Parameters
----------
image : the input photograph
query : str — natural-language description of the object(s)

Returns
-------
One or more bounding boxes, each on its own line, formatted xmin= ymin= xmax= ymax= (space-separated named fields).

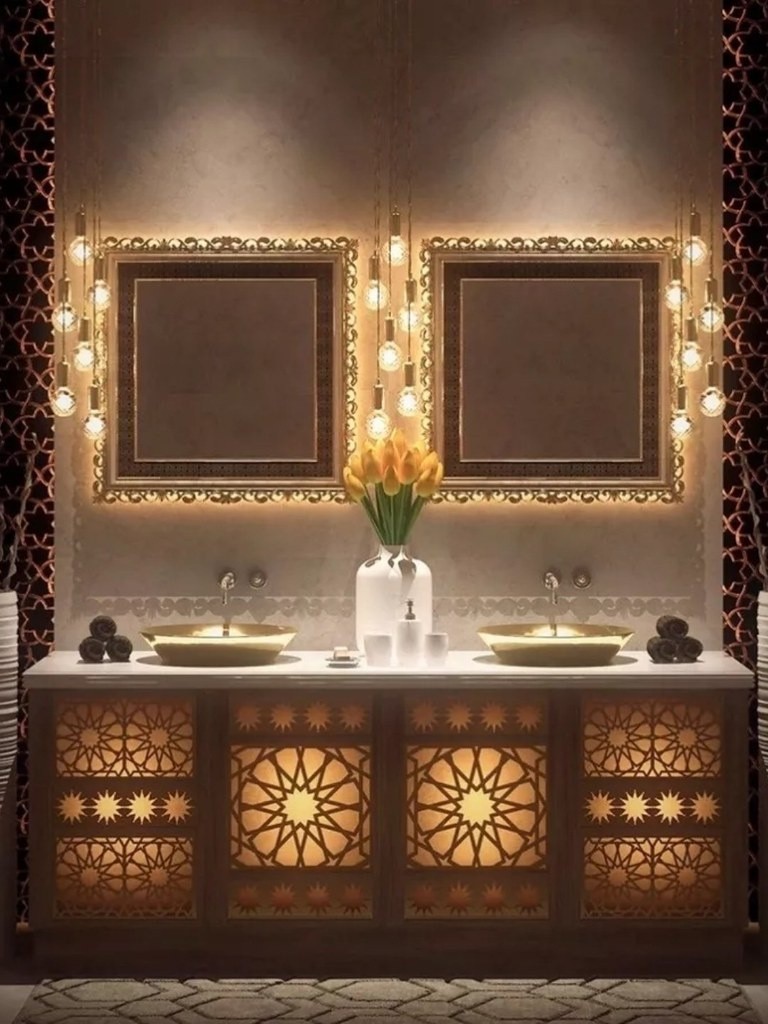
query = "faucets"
xmin=542 ymin=571 xmax=559 ymax=606
xmin=219 ymin=571 xmax=235 ymax=605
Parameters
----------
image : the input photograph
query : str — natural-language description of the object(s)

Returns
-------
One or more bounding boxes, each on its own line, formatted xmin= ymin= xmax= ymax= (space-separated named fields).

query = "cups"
xmin=425 ymin=633 xmax=448 ymax=668
xmin=364 ymin=633 xmax=392 ymax=666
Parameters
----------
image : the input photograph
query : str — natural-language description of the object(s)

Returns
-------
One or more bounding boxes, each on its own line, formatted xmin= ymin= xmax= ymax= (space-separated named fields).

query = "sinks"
xmin=138 ymin=622 xmax=300 ymax=668
xmin=475 ymin=622 xmax=637 ymax=668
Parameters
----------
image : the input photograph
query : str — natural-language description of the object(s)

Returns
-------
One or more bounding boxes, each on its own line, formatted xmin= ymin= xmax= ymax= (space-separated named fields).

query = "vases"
xmin=356 ymin=545 xmax=433 ymax=656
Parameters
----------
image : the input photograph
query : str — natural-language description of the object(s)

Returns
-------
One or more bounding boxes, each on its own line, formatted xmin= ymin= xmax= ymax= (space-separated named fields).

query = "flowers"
xmin=342 ymin=425 xmax=444 ymax=546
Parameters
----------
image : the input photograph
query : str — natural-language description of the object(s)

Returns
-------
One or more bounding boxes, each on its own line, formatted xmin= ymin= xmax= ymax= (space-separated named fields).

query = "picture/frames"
xmin=417 ymin=235 xmax=687 ymax=506
xmin=95 ymin=235 xmax=359 ymax=506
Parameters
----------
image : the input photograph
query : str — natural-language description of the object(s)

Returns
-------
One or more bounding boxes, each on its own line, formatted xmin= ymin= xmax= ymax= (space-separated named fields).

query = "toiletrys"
xmin=395 ymin=599 xmax=424 ymax=666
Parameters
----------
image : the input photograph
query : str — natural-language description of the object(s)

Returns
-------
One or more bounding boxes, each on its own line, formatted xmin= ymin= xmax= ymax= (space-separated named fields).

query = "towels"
xmin=78 ymin=615 xmax=134 ymax=663
xmin=646 ymin=615 xmax=703 ymax=663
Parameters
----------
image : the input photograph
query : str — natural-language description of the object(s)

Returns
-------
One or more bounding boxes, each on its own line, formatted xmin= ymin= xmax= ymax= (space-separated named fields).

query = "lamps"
xmin=51 ymin=0 xmax=114 ymax=439
xmin=664 ymin=0 xmax=725 ymax=436
xmin=362 ymin=0 xmax=424 ymax=440
